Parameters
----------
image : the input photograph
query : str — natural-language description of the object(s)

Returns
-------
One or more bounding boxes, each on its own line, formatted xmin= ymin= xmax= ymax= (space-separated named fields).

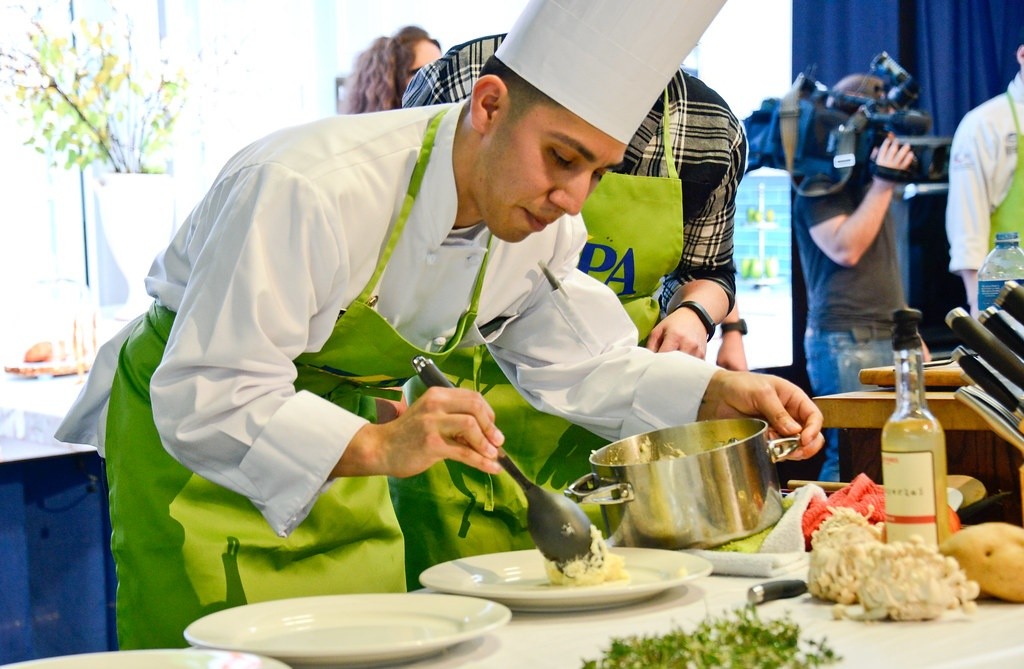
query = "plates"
xmin=420 ymin=544 xmax=714 ymax=612
xmin=183 ymin=593 xmax=512 ymax=668
xmin=1 ymin=647 xmax=294 ymax=668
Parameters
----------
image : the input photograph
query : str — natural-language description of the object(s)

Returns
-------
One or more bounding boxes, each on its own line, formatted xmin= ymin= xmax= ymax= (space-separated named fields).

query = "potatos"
xmin=938 ymin=522 xmax=1024 ymax=604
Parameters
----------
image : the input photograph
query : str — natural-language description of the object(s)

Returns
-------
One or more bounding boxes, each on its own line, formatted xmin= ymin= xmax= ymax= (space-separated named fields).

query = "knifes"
xmin=945 ymin=281 xmax=1024 ymax=460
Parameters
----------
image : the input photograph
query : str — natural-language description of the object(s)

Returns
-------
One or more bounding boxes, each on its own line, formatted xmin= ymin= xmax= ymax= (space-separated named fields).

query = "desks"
xmin=0 ymin=363 xmax=1024 ymax=669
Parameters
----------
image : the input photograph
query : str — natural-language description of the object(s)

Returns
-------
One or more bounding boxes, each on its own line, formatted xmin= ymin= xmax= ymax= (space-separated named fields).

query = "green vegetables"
xmin=576 ymin=603 xmax=845 ymax=669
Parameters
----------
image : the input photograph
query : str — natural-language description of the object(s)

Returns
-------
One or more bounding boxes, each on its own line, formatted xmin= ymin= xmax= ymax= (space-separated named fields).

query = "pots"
xmin=565 ymin=417 xmax=801 ymax=551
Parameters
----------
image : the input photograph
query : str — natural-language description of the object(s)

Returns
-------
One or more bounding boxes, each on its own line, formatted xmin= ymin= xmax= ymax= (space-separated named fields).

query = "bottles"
xmin=880 ymin=308 xmax=948 ymax=550
xmin=978 ymin=232 xmax=1024 ymax=314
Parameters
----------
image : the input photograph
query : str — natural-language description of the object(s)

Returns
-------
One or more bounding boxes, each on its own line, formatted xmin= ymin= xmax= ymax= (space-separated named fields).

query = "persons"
xmin=54 ymin=0 xmax=825 ymax=649
xmin=792 ymin=74 xmax=931 ymax=482
xmin=947 ymin=42 xmax=1024 ymax=318
xmin=343 ymin=26 xmax=748 ymax=591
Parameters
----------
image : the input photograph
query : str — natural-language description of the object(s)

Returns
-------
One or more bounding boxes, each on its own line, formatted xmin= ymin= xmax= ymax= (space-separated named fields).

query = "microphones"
xmin=870 ymin=111 xmax=931 ymax=136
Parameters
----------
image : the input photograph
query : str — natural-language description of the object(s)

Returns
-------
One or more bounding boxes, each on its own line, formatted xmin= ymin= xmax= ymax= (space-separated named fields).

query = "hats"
xmin=495 ymin=0 xmax=727 ymax=146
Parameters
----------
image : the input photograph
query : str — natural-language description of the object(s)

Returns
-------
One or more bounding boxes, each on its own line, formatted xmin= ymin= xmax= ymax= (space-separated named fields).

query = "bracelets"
xmin=674 ymin=300 xmax=715 ymax=341
xmin=722 ymin=318 xmax=748 ymax=335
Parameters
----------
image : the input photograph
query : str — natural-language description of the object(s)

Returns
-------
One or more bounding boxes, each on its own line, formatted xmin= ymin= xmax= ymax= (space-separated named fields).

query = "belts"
xmin=851 ymin=326 xmax=893 ymax=342
